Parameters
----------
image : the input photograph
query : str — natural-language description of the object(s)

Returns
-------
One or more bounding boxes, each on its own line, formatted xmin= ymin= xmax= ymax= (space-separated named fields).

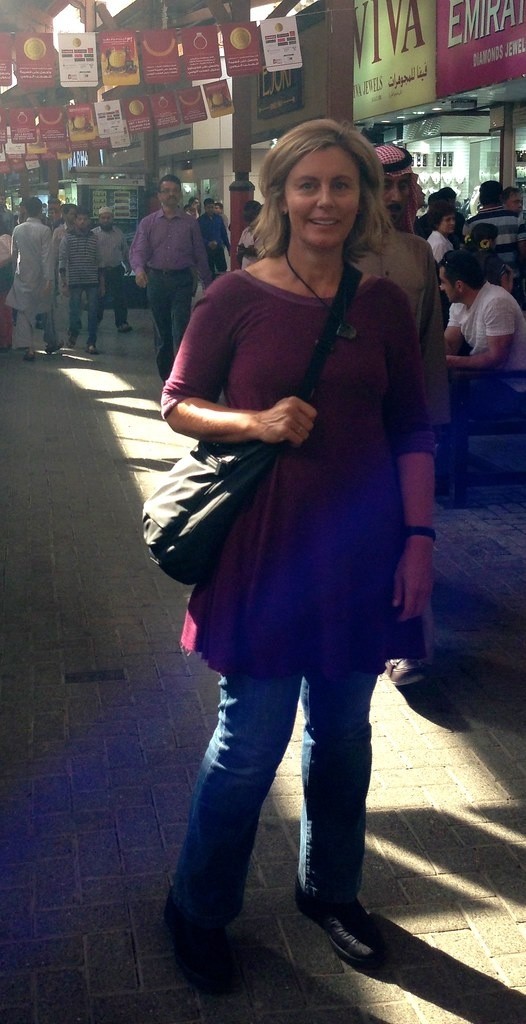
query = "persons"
xmin=461 ymin=181 xmax=526 ymax=310
xmin=415 ymin=187 xmax=465 ymax=240
xmin=6 ymin=197 xmax=63 ymax=360
xmin=503 ymin=187 xmax=523 ymax=212
xmin=236 ymin=200 xmax=262 ymax=270
xmin=161 ymin=119 xmax=436 ymax=993
xmin=214 ymin=203 xmax=229 ymax=229
xmin=92 ymin=207 xmax=132 ymax=332
xmin=130 ymin=174 xmax=212 ymax=387
xmin=184 ymin=197 xmax=200 ymax=220
xmin=197 ymin=198 xmax=230 ymax=279
xmin=52 ymin=203 xmax=77 ymax=348
xmin=437 ymin=250 xmax=526 ymax=509
xmin=59 ymin=205 xmax=99 ymax=354
xmin=426 ymin=200 xmax=456 ymax=263
xmin=467 ymin=224 xmax=513 ymax=292
xmin=349 ymin=148 xmax=451 ymax=687
xmin=0 ymin=192 xmax=65 ymax=352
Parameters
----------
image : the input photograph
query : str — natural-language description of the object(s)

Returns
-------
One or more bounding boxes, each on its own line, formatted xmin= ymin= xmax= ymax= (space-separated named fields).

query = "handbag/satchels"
xmin=142 ymin=439 xmax=273 ymax=583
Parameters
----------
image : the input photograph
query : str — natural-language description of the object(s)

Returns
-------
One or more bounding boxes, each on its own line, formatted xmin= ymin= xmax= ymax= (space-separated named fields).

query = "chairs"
xmin=442 ymin=370 xmax=526 ymax=507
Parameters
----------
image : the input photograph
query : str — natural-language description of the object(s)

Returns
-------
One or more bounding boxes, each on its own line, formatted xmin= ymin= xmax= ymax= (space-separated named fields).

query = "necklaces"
xmin=286 ymin=250 xmax=357 ymax=339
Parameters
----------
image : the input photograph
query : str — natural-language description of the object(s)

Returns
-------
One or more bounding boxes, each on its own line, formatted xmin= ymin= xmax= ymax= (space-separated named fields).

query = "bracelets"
xmin=404 ymin=526 xmax=436 ymax=543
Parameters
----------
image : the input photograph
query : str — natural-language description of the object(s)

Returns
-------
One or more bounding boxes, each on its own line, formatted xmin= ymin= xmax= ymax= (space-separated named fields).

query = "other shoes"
xmin=85 ymin=343 xmax=97 ymax=353
xmin=118 ymin=323 xmax=133 ymax=332
xmin=161 ymin=886 xmax=235 ymax=994
xmin=386 ymin=657 xmax=425 ymax=686
xmin=45 ymin=339 xmax=64 ymax=355
xmin=24 ymin=350 xmax=35 ymax=360
xmin=295 ymin=876 xmax=387 ymax=968
xmin=66 ymin=339 xmax=74 ymax=348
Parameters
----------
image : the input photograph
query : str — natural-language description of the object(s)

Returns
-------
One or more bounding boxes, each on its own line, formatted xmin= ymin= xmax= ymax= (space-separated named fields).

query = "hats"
xmin=99 ymin=207 xmax=112 ymax=215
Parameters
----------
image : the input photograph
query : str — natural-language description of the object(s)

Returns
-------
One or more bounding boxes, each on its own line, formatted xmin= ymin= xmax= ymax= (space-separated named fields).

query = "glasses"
xmin=160 ymin=188 xmax=178 ymax=194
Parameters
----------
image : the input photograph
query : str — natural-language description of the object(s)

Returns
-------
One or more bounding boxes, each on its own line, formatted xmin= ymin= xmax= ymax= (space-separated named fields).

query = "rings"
xmin=297 ymin=427 xmax=302 ymax=433
